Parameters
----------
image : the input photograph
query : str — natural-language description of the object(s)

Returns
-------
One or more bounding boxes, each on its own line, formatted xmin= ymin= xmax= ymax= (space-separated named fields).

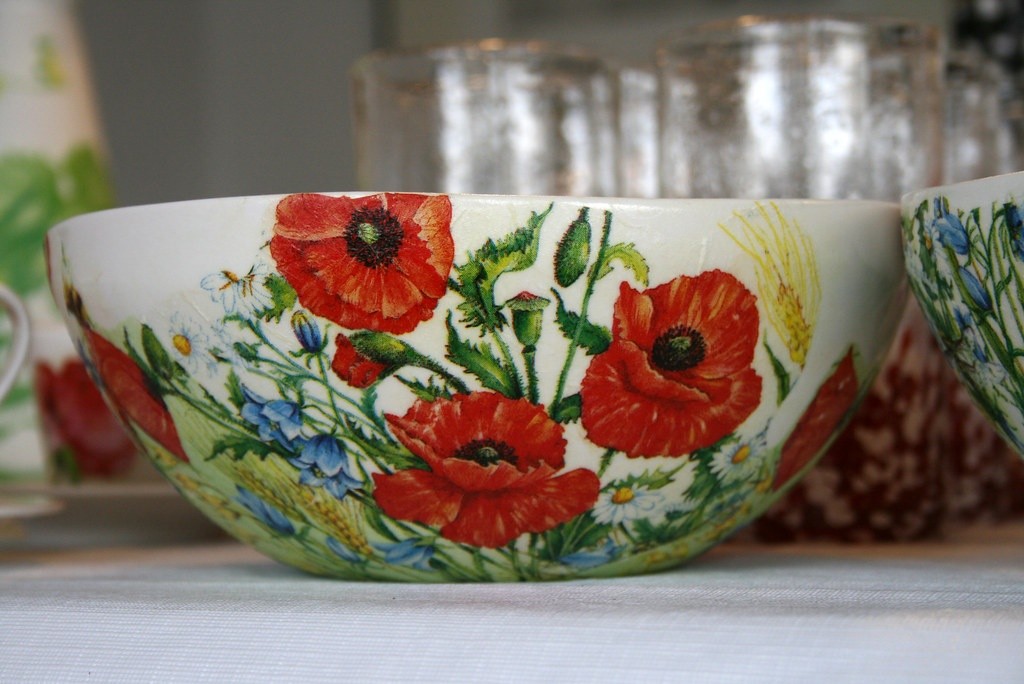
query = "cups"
xmin=350 ymin=15 xmax=1015 ymax=543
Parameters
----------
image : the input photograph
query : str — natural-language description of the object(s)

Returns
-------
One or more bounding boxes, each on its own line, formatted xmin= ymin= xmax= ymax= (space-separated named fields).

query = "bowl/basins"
xmin=42 ymin=190 xmax=913 ymax=585
xmin=900 ymin=169 xmax=1023 ymax=461
